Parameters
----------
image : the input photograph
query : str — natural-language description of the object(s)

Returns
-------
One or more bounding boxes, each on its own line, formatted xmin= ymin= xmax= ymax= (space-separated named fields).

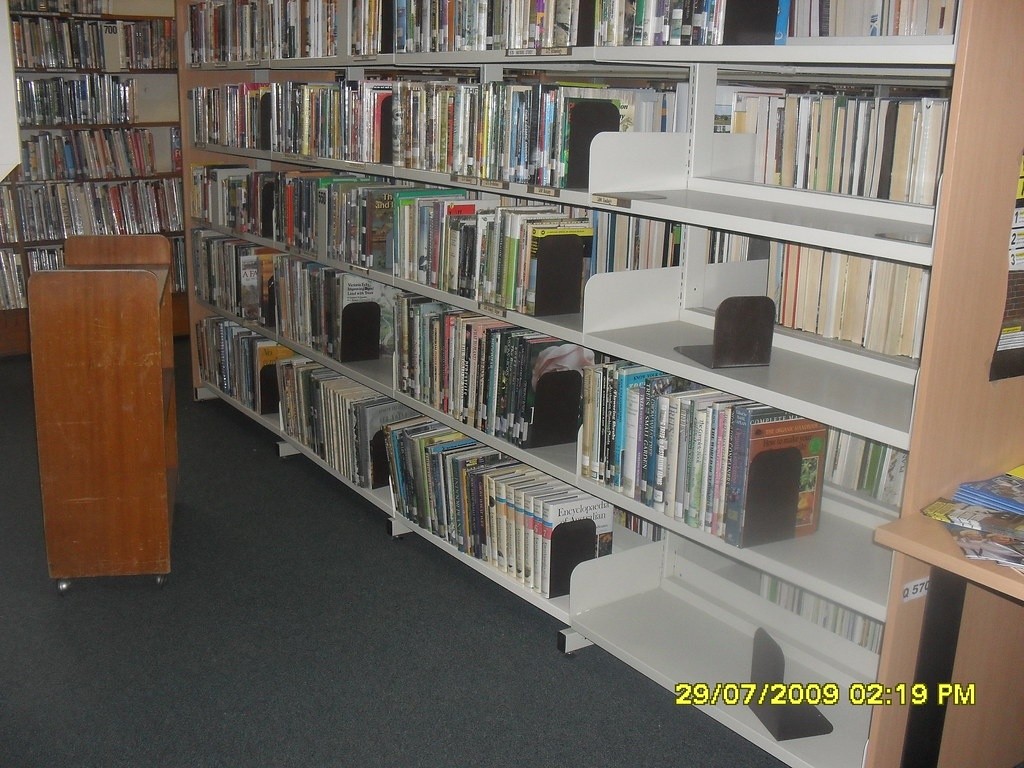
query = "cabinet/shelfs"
xmin=173 ymin=0 xmax=1024 ymax=768
xmin=0 ymin=0 xmax=187 ymax=359
xmin=862 ymin=0 xmax=1024 ymax=768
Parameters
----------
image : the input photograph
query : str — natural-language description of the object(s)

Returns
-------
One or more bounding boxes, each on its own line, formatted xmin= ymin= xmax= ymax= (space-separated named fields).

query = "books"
xmin=0 ymin=0 xmax=1024 ymax=598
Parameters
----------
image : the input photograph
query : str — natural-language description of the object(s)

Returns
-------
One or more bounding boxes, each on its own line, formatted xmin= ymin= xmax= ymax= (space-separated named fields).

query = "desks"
xmin=31 ymin=225 xmax=182 ymax=590
